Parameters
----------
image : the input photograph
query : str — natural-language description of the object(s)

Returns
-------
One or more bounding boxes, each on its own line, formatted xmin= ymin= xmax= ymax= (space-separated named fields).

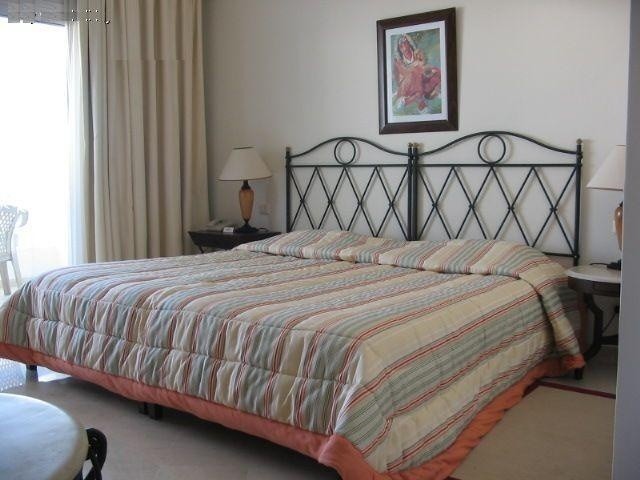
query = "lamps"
xmin=587 ymin=143 xmax=624 ymax=270
xmin=216 ymin=145 xmax=271 ymax=233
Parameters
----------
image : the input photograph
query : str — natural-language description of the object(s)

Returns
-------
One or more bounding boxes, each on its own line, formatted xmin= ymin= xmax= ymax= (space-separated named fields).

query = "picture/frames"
xmin=376 ymin=5 xmax=458 ymax=133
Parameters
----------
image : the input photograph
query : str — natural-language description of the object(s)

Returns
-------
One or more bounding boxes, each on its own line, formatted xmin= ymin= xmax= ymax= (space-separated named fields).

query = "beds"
xmin=24 ymin=132 xmax=581 ymax=475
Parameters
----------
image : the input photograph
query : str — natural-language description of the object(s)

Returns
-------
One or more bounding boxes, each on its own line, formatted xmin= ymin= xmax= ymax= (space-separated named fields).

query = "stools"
xmin=0 ymin=390 xmax=112 ymax=479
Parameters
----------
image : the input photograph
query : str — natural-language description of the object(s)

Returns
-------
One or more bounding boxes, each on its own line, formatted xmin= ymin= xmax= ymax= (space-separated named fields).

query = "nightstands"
xmin=188 ymin=225 xmax=282 ymax=258
xmin=568 ymin=262 xmax=619 ymax=378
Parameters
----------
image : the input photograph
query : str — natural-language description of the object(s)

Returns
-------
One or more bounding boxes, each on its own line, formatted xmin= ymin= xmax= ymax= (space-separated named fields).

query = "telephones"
xmin=203 ymin=218 xmax=236 ymax=232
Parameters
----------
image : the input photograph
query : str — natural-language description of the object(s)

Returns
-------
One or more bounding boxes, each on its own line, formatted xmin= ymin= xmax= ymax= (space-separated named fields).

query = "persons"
xmin=392 ymin=35 xmax=440 ymax=113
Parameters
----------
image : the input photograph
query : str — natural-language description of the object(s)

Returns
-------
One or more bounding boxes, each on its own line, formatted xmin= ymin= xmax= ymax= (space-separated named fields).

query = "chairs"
xmin=0 ymin=204 xmax=28 ymax=296
